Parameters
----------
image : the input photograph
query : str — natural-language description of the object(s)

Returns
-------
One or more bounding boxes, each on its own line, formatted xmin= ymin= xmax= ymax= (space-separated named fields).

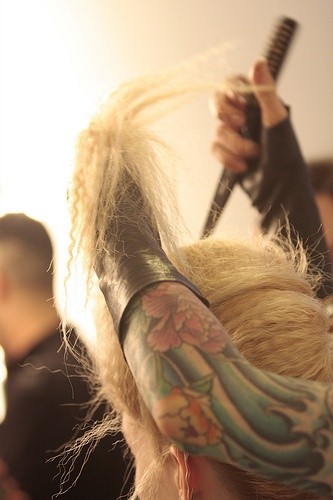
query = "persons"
xmin=86 ymin=57 xmax=333 ymax=498
xmin=92 ymin=240 xmax=333 ymax=500
xmin=0 ymin=211 xmax=134 ymax=500
xmin=306 ymin=158 xmax=333 ymax=259
